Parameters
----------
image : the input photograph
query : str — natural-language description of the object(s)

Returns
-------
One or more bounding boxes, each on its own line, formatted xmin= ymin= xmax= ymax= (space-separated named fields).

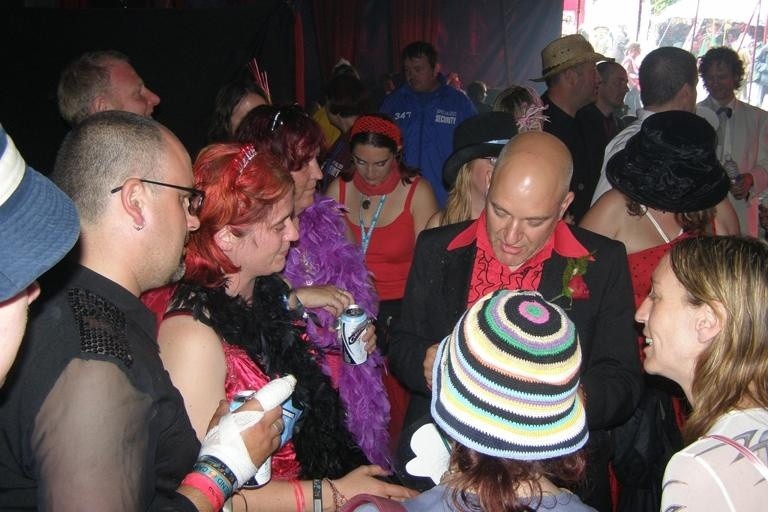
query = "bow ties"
xmin=717 ymin=107 xmax=732 ymax=118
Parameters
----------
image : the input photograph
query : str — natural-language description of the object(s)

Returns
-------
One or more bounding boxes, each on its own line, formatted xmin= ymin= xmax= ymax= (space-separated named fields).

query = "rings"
xmin=338 ymin=287 xmax=345 ymax=299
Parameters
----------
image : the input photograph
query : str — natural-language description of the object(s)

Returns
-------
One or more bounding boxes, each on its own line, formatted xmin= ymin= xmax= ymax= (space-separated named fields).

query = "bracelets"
xmin=325 ymin=477 xmax=348 ymax=511
xmin=221 ymin=491 xmax=248 ymax=512
xmin=291 ymin=477 xmax=322 ymax=512
xmin=181 ymin=453 xmax=237 ymax=512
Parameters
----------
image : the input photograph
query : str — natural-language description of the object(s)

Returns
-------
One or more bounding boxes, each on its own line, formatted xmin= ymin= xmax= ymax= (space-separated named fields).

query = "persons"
xmin=494 ymin=86 xmax=543 ymax=133
xmin=327 ymin=108 xmax=436 ymax=452
xmin=0 ymin=110 xmax=297 ymax=512
xmin=426 ymin=113 xmax=516 ymax=229
xmin=621 ymin=41 xmax=642 ymax=118
xmin=0 ymin=123 xmax=79 ymax=390
xmin=313 ymin=59 xmax=360 ymax=158
xmin=695 ymin=45 xmax=768 ymax=239
xmin=388 ymin=130 xmax=643 ymax=511
xmin=381 ymin=39 xmax=480 ymax=209
xmin=157 ymin=141 xmax=422 ymax=511
xmin=353 ymin=290 xmax=600 ymax=512
xmin=574 ymin=62 xmax=630 ymax=158
xmin=237 ymin=104 xmax=398 ymax=477
xmin=580 ymin=110 xmax=742 ymax=435
xmin=589 ymin=47 xmax=698 ymax=210
xmin=691 ymin=24 xmax=768 ymax=60
xmin=528 ymin=33 xmax=609 ymax=226
xmin=318 ymin=75 xmax=385 ymax=194
xmin=637 ymin=234 xmax=768 ymax=511
xmin=209 ymin=78 xmax=270 ymax=142
xmin=467 ymin=81 xmax=489 ymax=113
xmin=56 ymin=44 xmax=161 ymax=120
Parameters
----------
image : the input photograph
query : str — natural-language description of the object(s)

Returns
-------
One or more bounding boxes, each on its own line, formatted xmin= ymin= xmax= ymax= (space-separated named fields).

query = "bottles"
xmin=339 ymin=304 xmax=368 ymax=366
xmin=228 ymin=390 xmax=272 ymax=489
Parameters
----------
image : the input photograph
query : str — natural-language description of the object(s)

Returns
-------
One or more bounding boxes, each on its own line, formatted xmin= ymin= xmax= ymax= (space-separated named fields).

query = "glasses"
xmin=482 ymin=158 xmax=498 ymax=164
xmin=112 ymin=180 xmax=205 ymax=214
xmin=351 ymin=151 xmax=392 ymax=166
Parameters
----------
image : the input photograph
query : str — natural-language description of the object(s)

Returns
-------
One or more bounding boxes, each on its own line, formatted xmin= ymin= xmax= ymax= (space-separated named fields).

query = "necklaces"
xmin=362 ymin=192 xmax=372 ymax=211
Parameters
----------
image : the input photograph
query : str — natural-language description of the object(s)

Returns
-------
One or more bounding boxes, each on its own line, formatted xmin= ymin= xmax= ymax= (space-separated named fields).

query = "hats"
xmin=606 ymin=110 xmax=730 ymax=212
xmin=529 ymin=35 xmax=615 ymax=82
xmin=431 ymin=290 xmax=589 ymax=460
xmin=442 ymin=111 xmax=518 ymax=191
xmin=0 ymin=122 xmax=81 ymax=304
